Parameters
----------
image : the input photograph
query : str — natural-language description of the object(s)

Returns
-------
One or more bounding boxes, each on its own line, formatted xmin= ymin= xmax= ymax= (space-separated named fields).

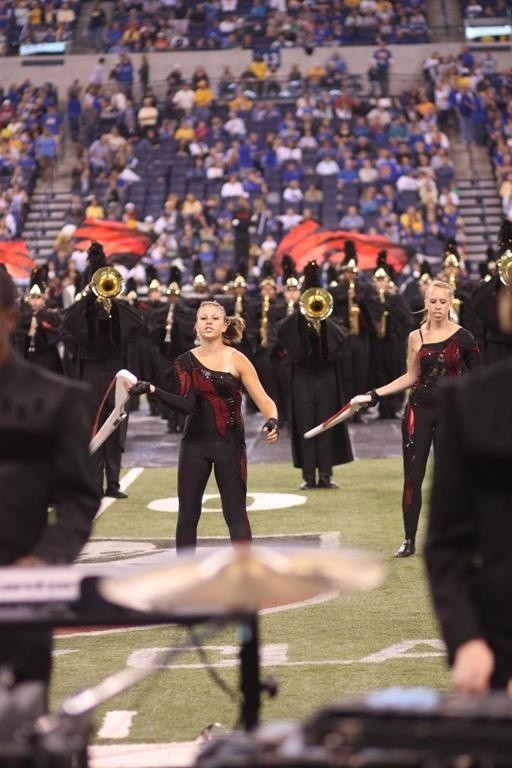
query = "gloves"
xmin=262 ymin=417 xmax=281 ymax=443
xmin=359 ymin=389 xmax=380 ymax=409
xmin=128 ymin=380 xmax=151 ymax=397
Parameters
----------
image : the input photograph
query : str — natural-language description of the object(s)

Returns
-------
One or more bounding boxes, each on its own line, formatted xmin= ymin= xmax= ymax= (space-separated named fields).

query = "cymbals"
xmin=96 ymin=546 xmax=388 ymax=621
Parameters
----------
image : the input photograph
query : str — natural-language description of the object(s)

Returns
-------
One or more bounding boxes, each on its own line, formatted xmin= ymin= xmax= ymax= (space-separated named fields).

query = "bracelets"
xmin=365 ymin=389 xmax=379 ymax=407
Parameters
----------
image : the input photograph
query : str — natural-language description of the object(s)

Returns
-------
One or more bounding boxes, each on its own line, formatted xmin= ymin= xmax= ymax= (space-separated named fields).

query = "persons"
xmin=16 ymin=240 xmax=497 ymax=499
xmin=464 ymin=0 xmax=509 ymax=19
xmin=49 ymin=40 xmax=465 ymax=286
xmin=1 ymin=78 xmax=63 ymax=239
xmin=421 ymin=244 xmax=512 ymax=696
xmin=105 ymin=1 xmax=433 ymax=53
xmin=123 ymin=298 xmax=282 ymax=559
xmin=350 ymin=279 xmax=484 ymax=557
xmin=0 ymin=262 xmax=103 ymax=704
xmin=0 ymin=1 xmax=77 ymax=56
xmin=422 ymin=46 xmax=512 ymax=253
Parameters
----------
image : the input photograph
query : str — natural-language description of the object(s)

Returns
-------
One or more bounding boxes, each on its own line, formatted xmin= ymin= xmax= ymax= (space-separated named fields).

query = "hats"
xmin=342 ymin=254 xmax=460 ymax=286
xmin=27 ymin=254 xmax=113 ymax=296
xmin=248 ymin=254 xmax=339 ymax=293
xmin=124 ymin=263 xmax=247 ymax=297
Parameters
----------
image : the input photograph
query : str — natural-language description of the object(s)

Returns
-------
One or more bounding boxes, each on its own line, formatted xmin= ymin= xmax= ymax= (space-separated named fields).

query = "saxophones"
xmin=347 ymin=279 xmax=360 ymax=335
xmin=260 ymin=295 xmax=269 ymax=349
xmin=376 ymin=290 xmax=389 ymax=340
xmin=447 ymin=275 xmax=461 ymax=326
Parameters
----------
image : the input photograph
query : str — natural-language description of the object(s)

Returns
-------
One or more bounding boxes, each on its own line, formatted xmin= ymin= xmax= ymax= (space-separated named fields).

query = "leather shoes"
xmin=300 ymin=482 xmax=317 ymax=488
xmin=318 ymin=481 xmax=336 ymax=488
xmin=105 ymin=488 xmax=129 ymax=499
xmin=394 ymin=539 xmax=416 ymax=557
